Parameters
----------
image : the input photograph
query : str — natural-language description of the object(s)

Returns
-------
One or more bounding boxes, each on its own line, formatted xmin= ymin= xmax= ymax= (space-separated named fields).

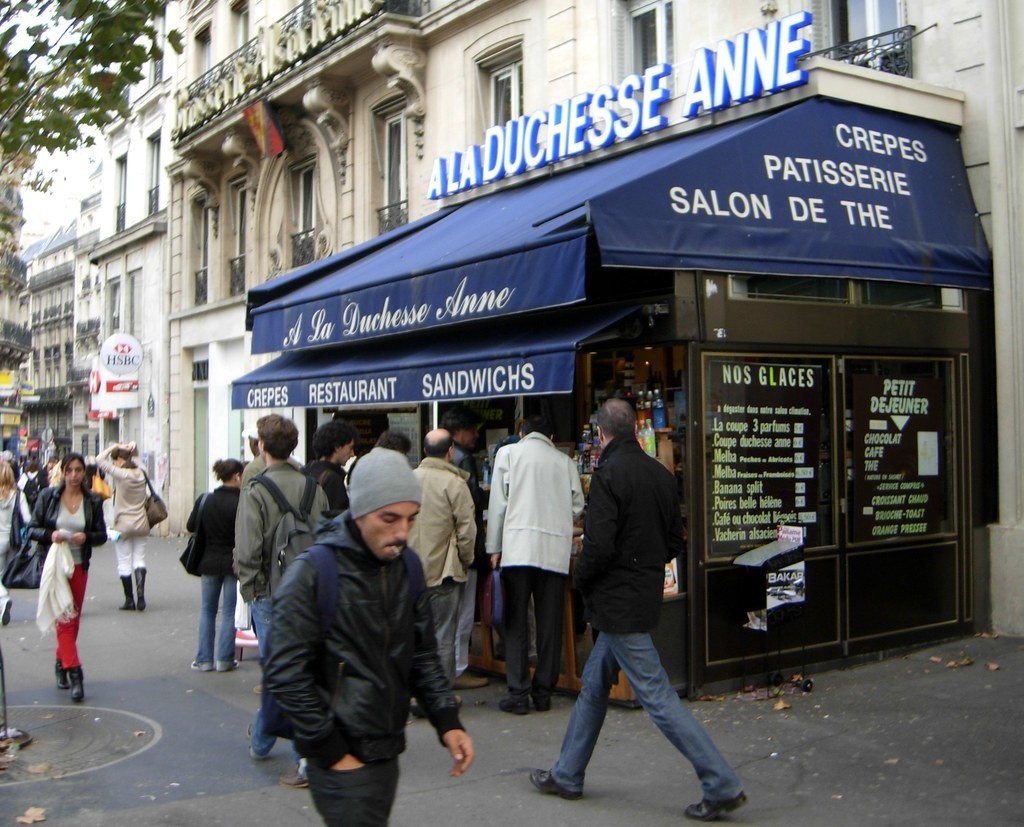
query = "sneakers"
xmin=530 ymin=768 xmax=583 ymax=800
xmin=686 ymin=785 xmax=746 ymax=821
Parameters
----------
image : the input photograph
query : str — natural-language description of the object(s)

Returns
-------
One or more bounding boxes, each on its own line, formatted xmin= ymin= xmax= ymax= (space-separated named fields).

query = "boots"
xmin=136 ymin=568 xmax=147 ymax=610
xmin=56 ymin=659 xmax=71 ymax=689
xmin=120 ymin=574 xmax=135 ymax=610
xmin=69 ymin=666 xmax=84 ymax=701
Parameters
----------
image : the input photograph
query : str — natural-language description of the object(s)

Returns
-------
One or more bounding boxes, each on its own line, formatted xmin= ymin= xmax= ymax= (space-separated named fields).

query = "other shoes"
xmin=217 ymin=660 xmax=240 ymax=671
xmin=247 ymin=718 xmax=268 ymax=760
xmin=1 ymin=598 xmax=12 ymax=624
xmin=191 ymin=660 xmax=212 ymax=671
xmin=279 ymin=772 xmax=311 ymax=788
xmin=452 ymin=671 xmax=489 ymax=688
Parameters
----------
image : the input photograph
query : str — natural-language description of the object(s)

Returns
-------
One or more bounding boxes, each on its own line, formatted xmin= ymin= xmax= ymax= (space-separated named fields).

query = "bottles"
xmin=481 ymin=457 xmax=491 ymax=485
xmin=572 ymin=369 xmax=667 ymax=474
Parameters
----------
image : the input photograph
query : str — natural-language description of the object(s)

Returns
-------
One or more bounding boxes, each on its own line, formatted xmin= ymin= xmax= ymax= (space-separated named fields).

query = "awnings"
xmin=230 ymin=298 xmax=658 ymax=409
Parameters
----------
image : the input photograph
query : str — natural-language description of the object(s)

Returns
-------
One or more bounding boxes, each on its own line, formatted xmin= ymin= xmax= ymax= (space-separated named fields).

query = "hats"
xmin=347 ymin=448 xmax=423 ymax=521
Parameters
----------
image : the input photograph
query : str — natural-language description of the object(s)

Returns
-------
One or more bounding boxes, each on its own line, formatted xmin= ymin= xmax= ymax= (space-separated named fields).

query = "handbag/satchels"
xmin=12 ymin=488 xmax=21 ymax=550
xmin=145 ymin=494 xmax=167 ymax=527
xmin=482 ymin=569 xmax=504 ymax=625
xmin=91 ymin=465 xmax=112 ymax=500
xmin=2 ymin=527 xmax=48 ymax=588
xmin=180 ymin=532 xmax=202 ymax=577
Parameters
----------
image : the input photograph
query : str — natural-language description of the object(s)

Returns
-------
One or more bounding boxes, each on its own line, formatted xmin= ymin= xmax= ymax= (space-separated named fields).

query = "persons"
xmin=186 ymin=459 xmax=245 ymax=672
xmin=231 ymin=415 xmax=489 ymax=789
xmin=529 ymin=398 xmax=747 ymax=820
xmin=0 ymin=448 xmax=59 ymax=548
xmin=264 ymin=447 xmax=475 ymax=827
xmin=84 ymin=454 xmax=105 ymax=506
xmin=26 ymin=453 xmax=107 ymax=700
xmin=485 ymin=415 xmax=584 ymax=714
xmin=0 ymin=462 xmax=31 ymax=626
xmin=95 ymin=441 xmax=150 ymax=611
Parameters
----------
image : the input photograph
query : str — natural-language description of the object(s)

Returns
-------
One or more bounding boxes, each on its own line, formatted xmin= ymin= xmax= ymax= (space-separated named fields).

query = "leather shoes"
xmin=500 ymin=698 xmax=529 ymax=714
xmin=537 ymin=701 xmax=550 ymax=711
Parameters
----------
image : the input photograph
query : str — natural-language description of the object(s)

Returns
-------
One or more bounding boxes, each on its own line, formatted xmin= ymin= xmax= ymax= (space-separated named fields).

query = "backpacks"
xmin=24 ymin=471 xmax=38 ymax=503
xmin=250 ymin=473 xmax=318 ymax=600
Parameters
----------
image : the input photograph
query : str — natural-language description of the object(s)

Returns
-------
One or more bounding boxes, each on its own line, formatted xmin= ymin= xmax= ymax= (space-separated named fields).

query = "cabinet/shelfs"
xmin=564 ymin=348 xmax=685 ymax=493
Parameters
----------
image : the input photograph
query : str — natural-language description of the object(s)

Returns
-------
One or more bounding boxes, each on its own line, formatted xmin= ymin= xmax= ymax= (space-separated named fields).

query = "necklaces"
xmin=66 ymin=498 xmax=80 ymax=508
